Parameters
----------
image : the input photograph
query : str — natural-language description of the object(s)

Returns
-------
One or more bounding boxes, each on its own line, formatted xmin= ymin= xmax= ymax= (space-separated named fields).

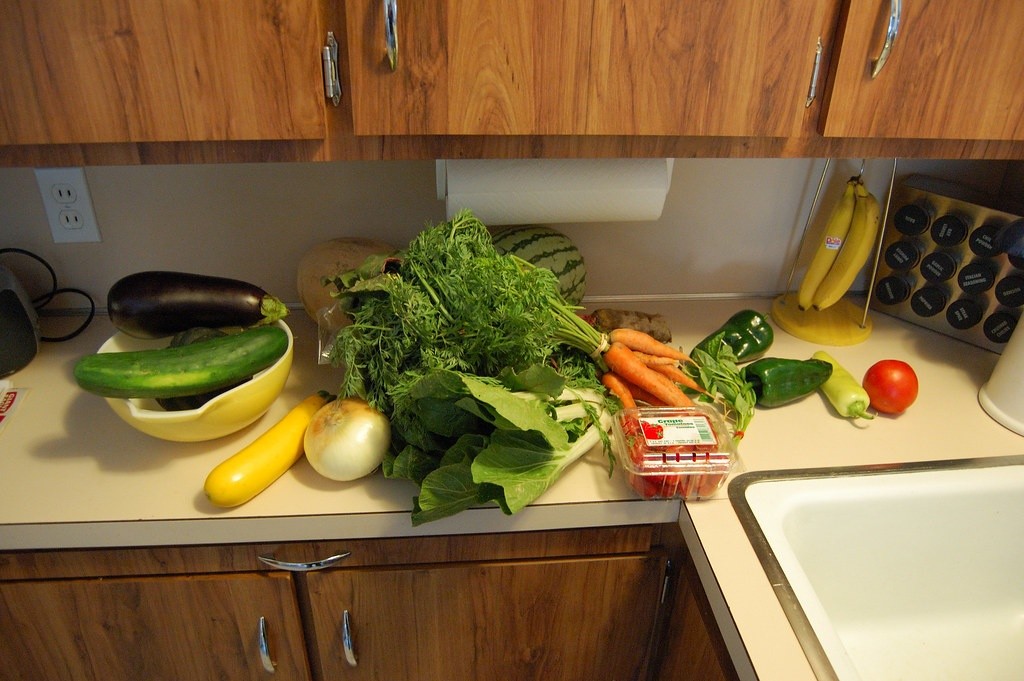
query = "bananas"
xmin=797 ymin=175 xmax=880 ymax=312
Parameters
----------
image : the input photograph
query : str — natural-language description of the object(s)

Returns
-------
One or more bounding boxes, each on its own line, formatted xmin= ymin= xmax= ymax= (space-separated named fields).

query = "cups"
xmin=979 ymin=311 xmax=1024 ymax=434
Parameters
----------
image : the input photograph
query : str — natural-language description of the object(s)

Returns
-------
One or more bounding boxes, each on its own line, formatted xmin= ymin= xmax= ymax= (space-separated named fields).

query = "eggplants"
xmin=106 ymin=270 xmax=291 ymax=339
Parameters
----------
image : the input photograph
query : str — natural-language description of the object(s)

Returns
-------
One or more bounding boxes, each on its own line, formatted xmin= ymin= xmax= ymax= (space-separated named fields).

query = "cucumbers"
xmin=72 ymin=325 xmax=290 ymax=396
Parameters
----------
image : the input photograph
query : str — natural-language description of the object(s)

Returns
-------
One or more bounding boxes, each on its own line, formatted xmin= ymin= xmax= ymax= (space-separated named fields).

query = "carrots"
xmin=599 ymin=327 xmax=724 ymax=418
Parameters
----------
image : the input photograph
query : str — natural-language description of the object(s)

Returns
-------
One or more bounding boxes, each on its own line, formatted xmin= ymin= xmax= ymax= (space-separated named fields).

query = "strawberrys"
xmin=617 ymin=410 xmax=727 ymax=500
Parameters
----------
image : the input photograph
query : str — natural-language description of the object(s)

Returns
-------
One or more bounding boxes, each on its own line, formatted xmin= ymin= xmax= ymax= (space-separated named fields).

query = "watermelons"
xmin=486 ymin=225 xmax=589 ymax=308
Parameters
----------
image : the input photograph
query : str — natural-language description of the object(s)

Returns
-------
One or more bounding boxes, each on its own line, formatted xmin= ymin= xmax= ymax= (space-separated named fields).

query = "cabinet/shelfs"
xmin=0 ymin=0 xmax=1024 ymax=168
xmin=0 ymin=522 xmax=741 ymax=681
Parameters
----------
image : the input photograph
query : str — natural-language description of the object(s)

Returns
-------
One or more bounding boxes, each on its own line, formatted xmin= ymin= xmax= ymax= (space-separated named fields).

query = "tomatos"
xmin=862 ymin=359 xmax=918 ymax=414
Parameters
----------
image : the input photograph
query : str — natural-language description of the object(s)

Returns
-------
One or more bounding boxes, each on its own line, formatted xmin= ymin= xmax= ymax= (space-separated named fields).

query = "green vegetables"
xmin=673 ymin=330 xmax=756 ymax=448
xmin=371 ymin=363 xmax=613 ymax=528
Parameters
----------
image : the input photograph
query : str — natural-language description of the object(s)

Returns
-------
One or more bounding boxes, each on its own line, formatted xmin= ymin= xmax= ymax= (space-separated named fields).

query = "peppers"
xmin=690 ymin=309 xmax=774 ymax=365
xmin=739 ymin=357 xmax=833 ymax=407
xmin=812 ymin=351 xmax=878 ymax=420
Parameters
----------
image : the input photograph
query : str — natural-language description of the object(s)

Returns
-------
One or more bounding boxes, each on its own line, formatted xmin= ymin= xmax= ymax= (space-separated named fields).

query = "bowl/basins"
xmin=97 ymin=319 xmax=294 ymax=442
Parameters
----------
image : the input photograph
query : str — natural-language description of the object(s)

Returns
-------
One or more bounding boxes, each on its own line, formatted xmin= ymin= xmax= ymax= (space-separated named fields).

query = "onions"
xmin=304 ymin=397 xmax=392 ymax=482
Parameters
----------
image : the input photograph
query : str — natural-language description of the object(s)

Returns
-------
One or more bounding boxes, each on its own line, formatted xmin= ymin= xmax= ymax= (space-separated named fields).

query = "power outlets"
xmin=34 ymin=166 xmax=103 ymax=244
